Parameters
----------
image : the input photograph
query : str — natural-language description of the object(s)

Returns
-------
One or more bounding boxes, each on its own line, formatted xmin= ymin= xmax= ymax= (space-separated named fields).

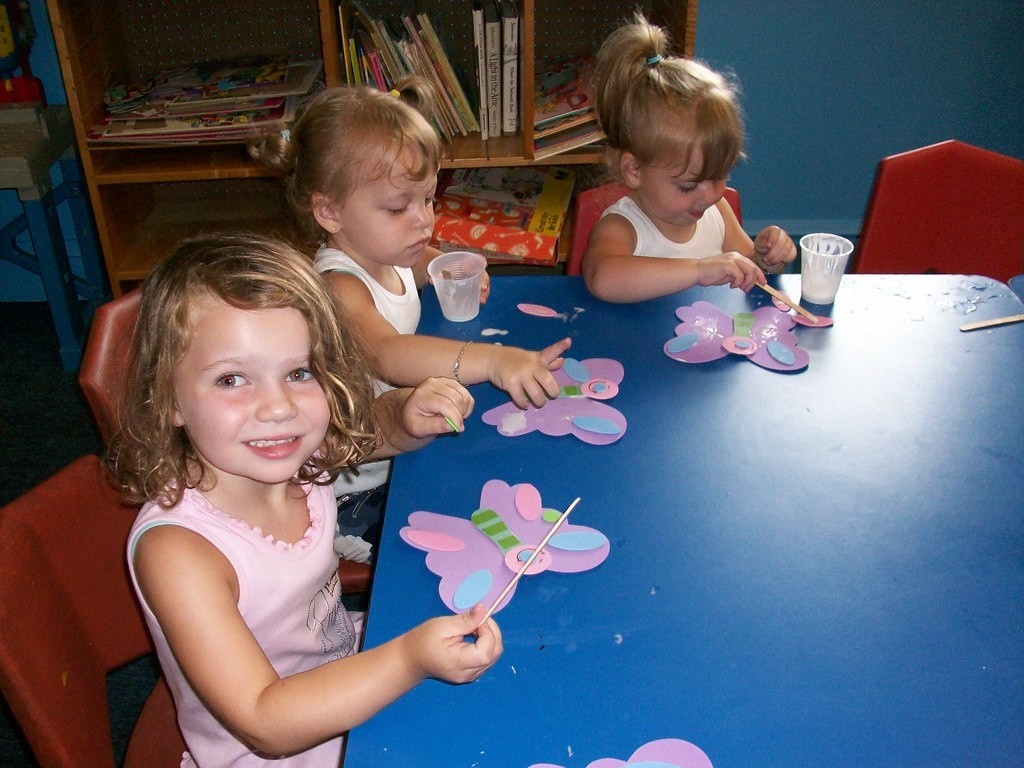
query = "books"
xmin=337 ymin=0 xmax=519 ymax=146
xmin=91 ymin=55 xmax=324 ymax=141
xmin=535 ymin=53 xmax=608 ymax=161
xmin=428 ymin=166 xmax=575 ymax=267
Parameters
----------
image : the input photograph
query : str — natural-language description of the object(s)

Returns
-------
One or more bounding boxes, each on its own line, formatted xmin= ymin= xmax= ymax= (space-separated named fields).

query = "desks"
xmin=0 ymin=103 xmax=110 ymax=376
xmin=343 ymin=271 xmax=1024 ymax=768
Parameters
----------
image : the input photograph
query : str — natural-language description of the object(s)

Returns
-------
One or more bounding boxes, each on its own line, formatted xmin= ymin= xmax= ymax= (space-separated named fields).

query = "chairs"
xmin=564 ymin=182 xmax=742 ymax=276
xmin=0 ymin=453 xmax=189 ymax=768
xmin=77 ymin=288 xmax=374 ymax=597
xmin=846 ymin=138 xmax=1024 ymax=283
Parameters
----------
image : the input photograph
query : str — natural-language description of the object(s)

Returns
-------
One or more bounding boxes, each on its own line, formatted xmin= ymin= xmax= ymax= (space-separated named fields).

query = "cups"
xmin=799 ymin=233 xmax=854 ymax=305
xmin=427 ymin=251 xmax=487 ymax=322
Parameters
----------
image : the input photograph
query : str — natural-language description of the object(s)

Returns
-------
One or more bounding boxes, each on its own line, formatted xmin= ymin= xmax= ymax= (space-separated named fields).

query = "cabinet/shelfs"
xmin=46 ymin=0 xmax=699 ymax=299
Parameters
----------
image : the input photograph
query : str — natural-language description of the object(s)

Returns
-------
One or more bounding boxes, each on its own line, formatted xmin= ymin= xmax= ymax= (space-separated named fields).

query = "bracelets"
xmin=452 ymin=341 xmax=473 ymax=388
xmin=752 ymin=251 xmax=786 ymax=275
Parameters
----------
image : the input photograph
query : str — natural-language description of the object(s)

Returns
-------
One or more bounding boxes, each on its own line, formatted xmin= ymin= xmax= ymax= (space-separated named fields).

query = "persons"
xmin=249 ymin=84 xmax=572 ymax=567
xmin=580 ymin=10 xmax=798 ymax=303
xmin=101 ymin=228 xmax=504 ymax=768
